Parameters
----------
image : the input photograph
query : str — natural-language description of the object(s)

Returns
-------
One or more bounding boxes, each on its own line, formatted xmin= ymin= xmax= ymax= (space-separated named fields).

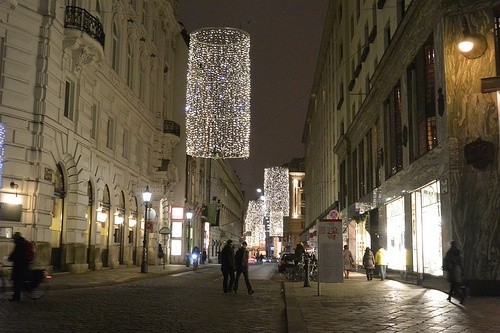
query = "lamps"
xmin=458 ymin=33 xmax=488 ymax=59
xmin=10 ymin=182 xmax=22 ymax=205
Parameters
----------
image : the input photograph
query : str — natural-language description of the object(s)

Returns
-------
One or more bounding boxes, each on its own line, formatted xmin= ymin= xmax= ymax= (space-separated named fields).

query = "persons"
xmin=7 ymin=231 xmax=35 ymax=302
xmin=234 ymin=241 xmax=255 ymax=295
xmin=256 ymin=253 xmax=263 ymax=263
xmin=221 ymin=238 xmax=235 ymax=294
xmin=201 ymin=250 xmax=207 ymax=264
xmin=374 ymin=244 xmax=389 ymax=281
xmin=342 ymin=245 xmax=354 ymax=279
xmin=442 ymin=240 xmax=466 ymax=304
xmin=363 ymin=248 xmax=375 ymax=281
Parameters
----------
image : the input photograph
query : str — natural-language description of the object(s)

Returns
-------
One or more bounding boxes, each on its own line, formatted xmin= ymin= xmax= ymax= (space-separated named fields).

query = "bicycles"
xmin=285 ymin=260 xmax=318 ymax=282
xmin=1 ymin=263 xmax=51 ymax=300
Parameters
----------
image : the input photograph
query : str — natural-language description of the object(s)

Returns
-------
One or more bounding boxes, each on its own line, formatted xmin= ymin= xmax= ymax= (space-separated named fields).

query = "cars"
xmin=277 ymin=252 xmax=296 ymax=272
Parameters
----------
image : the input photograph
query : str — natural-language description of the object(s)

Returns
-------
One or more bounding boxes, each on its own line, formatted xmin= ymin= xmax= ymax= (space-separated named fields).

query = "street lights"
xmin=185 ymin=208 xmax=194 ymax=267
xmin=140 ymin=185 xmax=153 ymax=273
